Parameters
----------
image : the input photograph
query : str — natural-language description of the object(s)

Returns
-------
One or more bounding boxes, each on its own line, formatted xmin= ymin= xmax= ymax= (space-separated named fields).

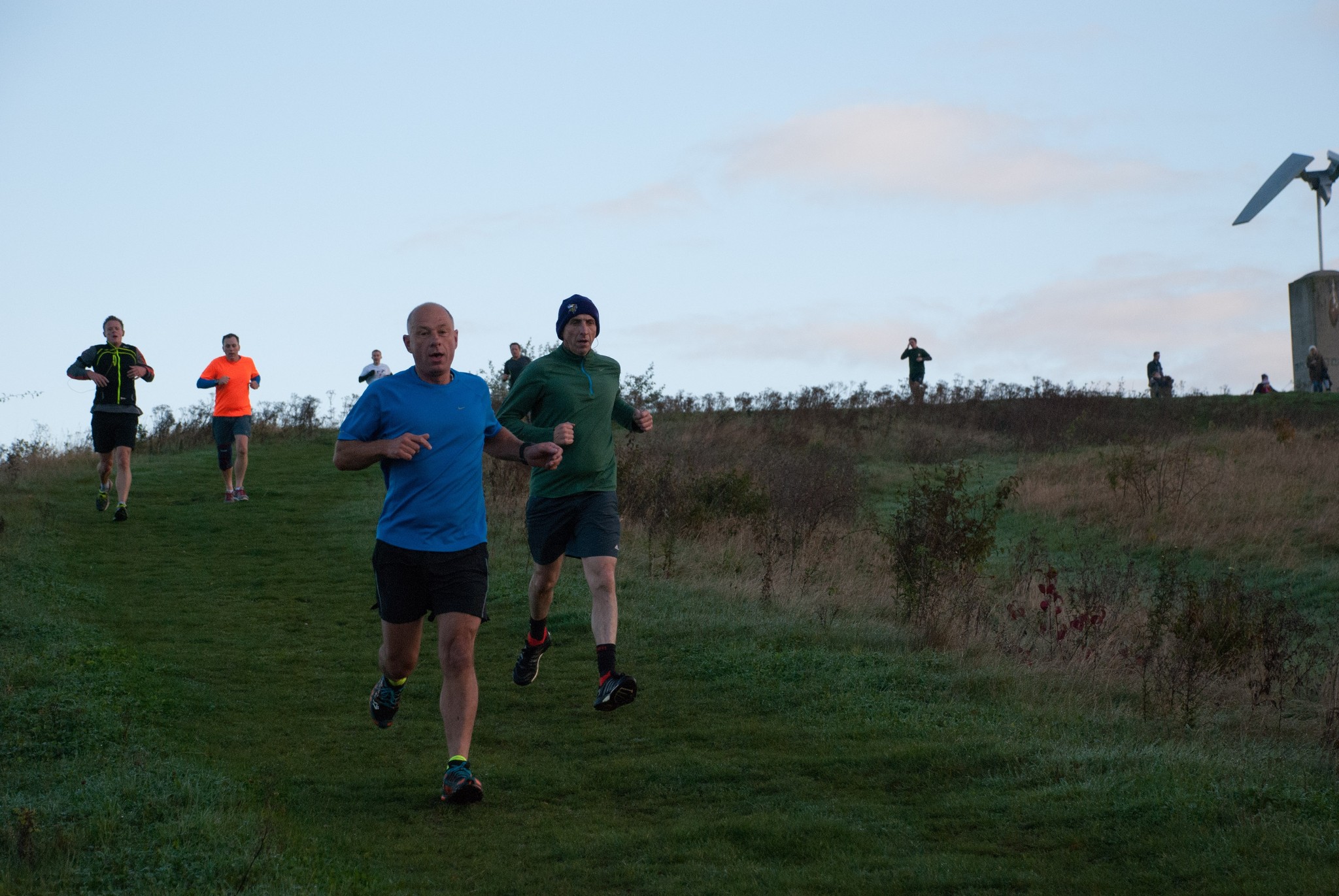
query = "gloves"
xmin=368 ymin=370 xmax=375 ymax=377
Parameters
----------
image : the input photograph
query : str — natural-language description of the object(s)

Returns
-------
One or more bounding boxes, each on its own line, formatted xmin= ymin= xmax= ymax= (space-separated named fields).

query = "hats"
xmin=556 ymin=294 xmax=600 ymax=341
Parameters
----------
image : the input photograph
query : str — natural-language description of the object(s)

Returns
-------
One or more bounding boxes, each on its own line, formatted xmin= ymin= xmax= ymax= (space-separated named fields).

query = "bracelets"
xmin=518 ymin=441 xmax=537 ymax=466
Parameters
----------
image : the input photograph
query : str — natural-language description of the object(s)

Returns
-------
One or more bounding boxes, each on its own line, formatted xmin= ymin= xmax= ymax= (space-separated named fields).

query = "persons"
xmin=196 ymin=332 xmax=261 ymax=506
xmin=66 ymin=315 xmax=155 ymax=522
xmin=358 ymin=349 xmax=393 ymax=386
xmin=502 ymin=342 xmax=531 ymax=392
xmin=497 ymin=294 xmax=653 ymax=714
xmin=1147 ymin=351 xmax=1174 ymax=400
xmin=899 ymin=337 xmax=932 ymax=396
xmin=1254 ymin=374 xmax=1277 ymax=394
xmin=329 ymin=301 xmax=564 ymax=805
xmin=1305 ymin=345 xmax=1333 ymax=393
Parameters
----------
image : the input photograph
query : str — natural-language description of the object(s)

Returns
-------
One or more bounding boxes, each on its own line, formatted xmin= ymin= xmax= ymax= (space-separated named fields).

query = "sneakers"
xmin=594 ymin=670 xmax=638 ymax=712
xmin=234 ymin=486 xmax=249 ymax=501
xmin=440 ymin=759 xmax=484 ymax=804
xmin=223 ymin=485 xmax=236 ymax=503
xmin=369 ymin=675 xmax=408 ymax=728
xmin=513 ymin=631 xmax=553 ymax=686
xmin=113 ymin=503 xmax=128 ymax=521
xmin=96 ymin=479 xmax=113 ymax=511
